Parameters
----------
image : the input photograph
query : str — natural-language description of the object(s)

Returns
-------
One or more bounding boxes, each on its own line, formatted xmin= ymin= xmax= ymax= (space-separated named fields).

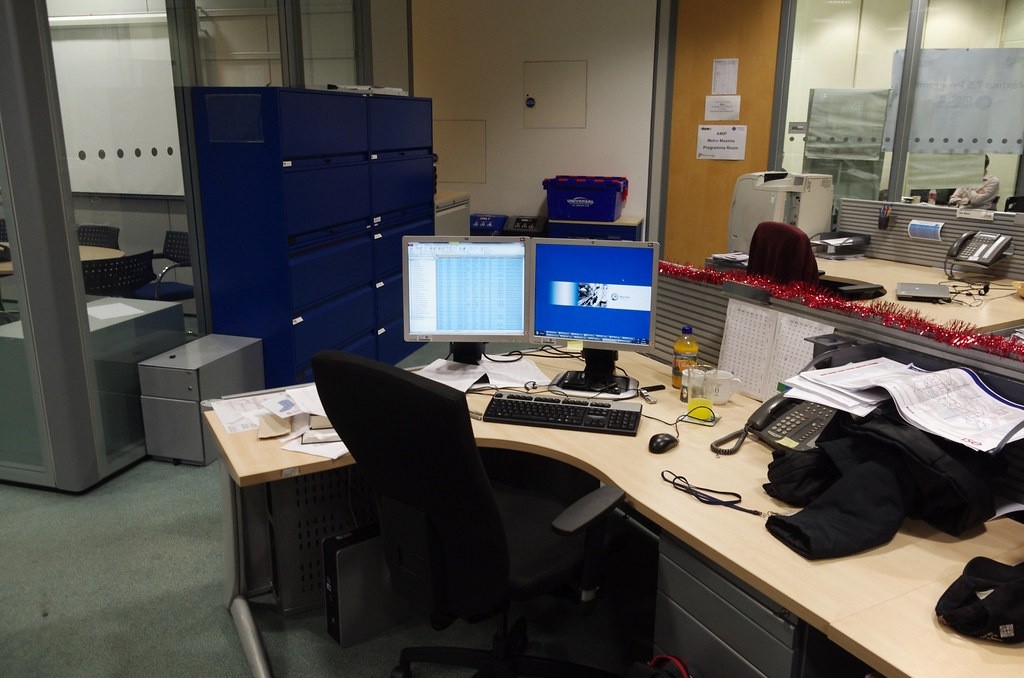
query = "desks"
xmin=0 ymin=242 xmax=125 ymax=324
xmin=705 ymin=250 xmax=1024 ymax=339
xmin=201 ymin=340 xmax=1024 ymax=678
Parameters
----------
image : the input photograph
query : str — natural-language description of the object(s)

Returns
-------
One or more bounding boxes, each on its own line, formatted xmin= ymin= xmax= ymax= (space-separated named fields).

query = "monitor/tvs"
xmin=403 ymin=235 xmax=660 ymax=400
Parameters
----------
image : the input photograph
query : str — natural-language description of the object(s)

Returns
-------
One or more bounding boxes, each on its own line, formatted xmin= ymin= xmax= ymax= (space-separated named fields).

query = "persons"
xmin=948 ymin=154 xmax=1000 ymax=210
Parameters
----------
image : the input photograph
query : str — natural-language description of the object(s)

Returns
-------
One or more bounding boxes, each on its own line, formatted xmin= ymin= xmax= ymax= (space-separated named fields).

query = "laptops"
xmin=896 ymin=283 xmax=951 ymax=303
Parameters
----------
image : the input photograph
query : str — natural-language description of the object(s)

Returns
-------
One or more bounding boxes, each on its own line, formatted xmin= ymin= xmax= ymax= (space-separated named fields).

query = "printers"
xmin=728 ymin=171 xmax=833 ymax=253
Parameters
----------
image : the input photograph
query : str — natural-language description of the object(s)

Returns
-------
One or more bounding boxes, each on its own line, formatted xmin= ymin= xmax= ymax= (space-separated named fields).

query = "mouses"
xmin=649 ymin=433 xmax=679 ymax=454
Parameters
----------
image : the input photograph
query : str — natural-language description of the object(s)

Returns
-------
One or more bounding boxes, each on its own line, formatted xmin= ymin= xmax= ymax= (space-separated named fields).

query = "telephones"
xmin=745 ymin=387 xmax=838 ymax=452
xmin=947 ymin=231 xmax=1013 ymax=266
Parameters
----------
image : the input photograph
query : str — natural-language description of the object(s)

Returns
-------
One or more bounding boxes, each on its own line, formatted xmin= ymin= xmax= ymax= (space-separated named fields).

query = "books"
xmin=777 ymin=356 xmax=1024 ymax=454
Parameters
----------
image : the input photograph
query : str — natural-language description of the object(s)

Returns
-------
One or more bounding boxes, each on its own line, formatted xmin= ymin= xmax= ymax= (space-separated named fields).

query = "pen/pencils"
xmin=879 ymin=204 xmax=892 ymax=216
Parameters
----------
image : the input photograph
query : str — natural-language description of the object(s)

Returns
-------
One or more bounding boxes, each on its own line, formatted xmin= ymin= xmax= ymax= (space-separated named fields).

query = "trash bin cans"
xmin=470 ymin=213 xmax=507 ymax=236
xmin=502 ymin=215 xmax=548 ymax=237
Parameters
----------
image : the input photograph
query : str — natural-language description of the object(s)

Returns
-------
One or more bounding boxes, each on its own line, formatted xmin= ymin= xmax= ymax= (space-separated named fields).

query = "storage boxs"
xmin=139 ymin=334 xmax=265 ymax=465
xmin=541 ymin=174 xmax=628 ymax=222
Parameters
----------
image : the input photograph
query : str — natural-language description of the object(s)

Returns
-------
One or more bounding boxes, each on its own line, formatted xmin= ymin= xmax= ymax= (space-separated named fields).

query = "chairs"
xmin=747 ymin=221 xmax=884 ymax=302
xmin=306 ymin=350 xmax=654 ymax=678
xmin=0 ymin=218 xmax=199 ymax=340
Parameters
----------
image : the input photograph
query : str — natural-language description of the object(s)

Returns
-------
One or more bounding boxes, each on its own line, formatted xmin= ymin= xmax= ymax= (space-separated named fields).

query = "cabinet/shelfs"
xmin=191 ymin=85 xmax=437 ymax=394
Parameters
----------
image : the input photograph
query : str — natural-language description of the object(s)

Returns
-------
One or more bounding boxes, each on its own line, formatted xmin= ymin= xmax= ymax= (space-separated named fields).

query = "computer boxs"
xmin=321 ymin=522 xmax=414 ymax=648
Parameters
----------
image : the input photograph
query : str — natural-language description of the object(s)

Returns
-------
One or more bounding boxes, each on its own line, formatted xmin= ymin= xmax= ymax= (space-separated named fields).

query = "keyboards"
xmin=482 ymin=391 xmax=642 ymax=436
